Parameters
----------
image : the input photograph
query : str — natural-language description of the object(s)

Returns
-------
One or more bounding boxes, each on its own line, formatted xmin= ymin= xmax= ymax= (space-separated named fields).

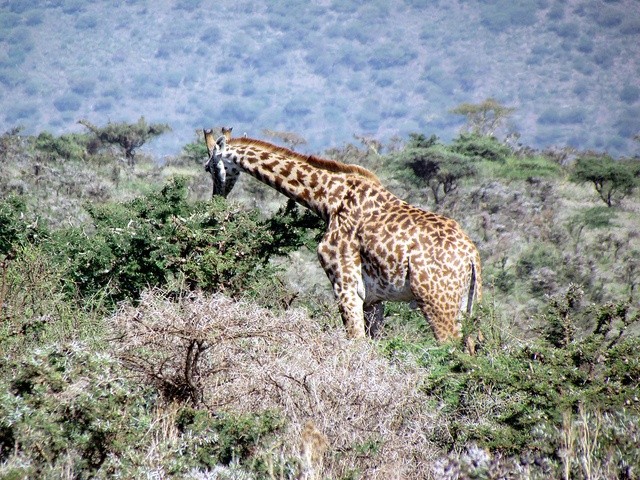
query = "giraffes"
xmin=202 ymin=127 xmax=484 ymax=362
